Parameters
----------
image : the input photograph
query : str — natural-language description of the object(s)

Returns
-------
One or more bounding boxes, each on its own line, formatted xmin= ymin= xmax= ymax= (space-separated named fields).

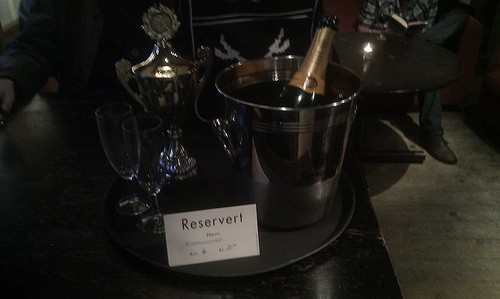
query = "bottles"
xmin=278 ymin=14 xmax=339 ymax=109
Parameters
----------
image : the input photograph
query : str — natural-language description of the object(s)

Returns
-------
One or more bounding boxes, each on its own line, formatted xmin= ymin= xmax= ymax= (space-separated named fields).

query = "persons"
xmin=1 ymin=0 xmax=476 ymax=168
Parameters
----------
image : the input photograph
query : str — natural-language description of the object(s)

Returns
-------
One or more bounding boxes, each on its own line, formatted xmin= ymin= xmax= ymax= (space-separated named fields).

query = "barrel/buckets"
xmin=208 ymin=56 xmax=362 ymax=229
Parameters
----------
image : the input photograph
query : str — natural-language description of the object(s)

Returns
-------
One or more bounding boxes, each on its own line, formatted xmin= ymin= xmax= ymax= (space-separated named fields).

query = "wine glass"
xmin=377 ymin=3 xmax=391 ymax=42
xmin=121 ymin=115 xmax=174 ymax=234
xmin=95 ymin=102 xmax=154 ymax=217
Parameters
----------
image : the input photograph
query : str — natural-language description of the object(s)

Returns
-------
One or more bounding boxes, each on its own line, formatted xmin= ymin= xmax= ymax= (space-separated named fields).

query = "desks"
xmin=328 ymin=32 xmax=464 ymax=192
xmin=0 ymin=33 xmax=405 ymax=299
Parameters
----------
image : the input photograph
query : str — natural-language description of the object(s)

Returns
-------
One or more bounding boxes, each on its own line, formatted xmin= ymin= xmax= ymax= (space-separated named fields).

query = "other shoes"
xmin=420 ymin=128 xmax=457 ymax=165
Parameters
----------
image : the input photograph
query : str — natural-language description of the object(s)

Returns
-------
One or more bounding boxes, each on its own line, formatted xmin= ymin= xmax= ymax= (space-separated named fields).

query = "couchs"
xmin=323 ymin=0 xmax=484 ymax=109
